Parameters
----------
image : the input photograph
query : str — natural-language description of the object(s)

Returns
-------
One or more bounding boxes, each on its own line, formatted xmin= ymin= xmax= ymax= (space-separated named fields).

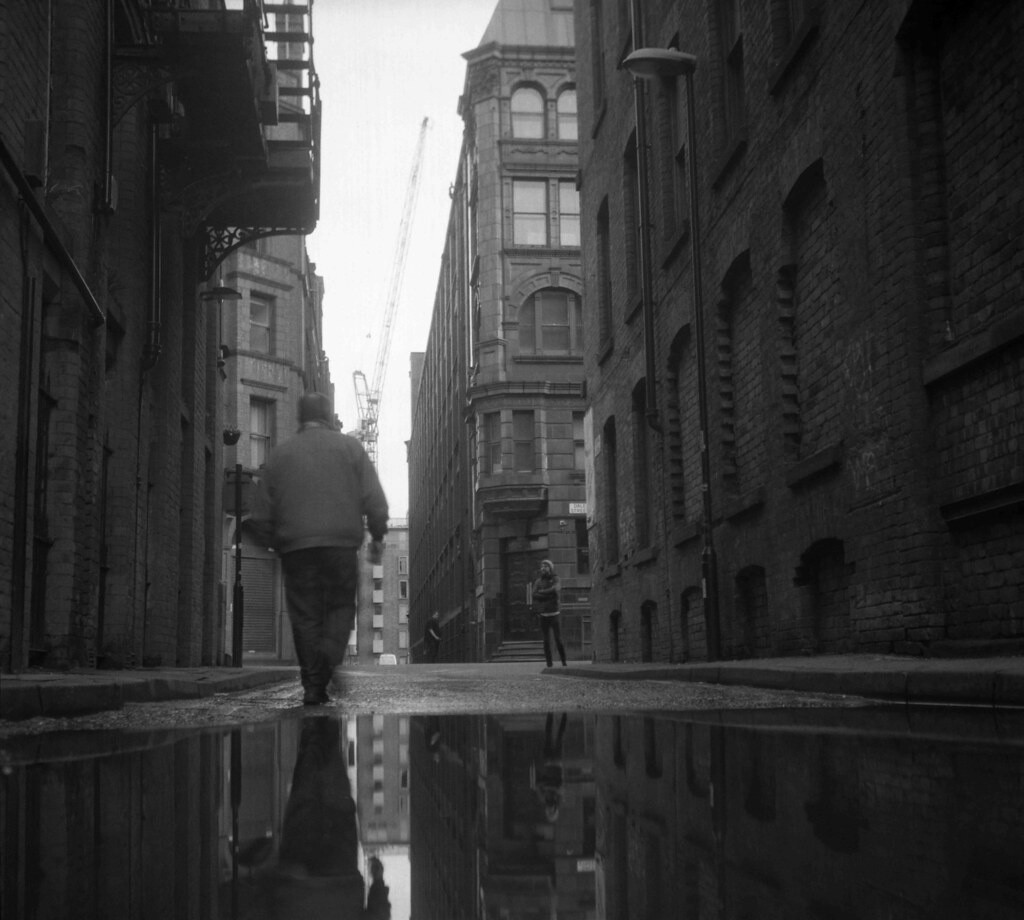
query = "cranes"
xmin=347 ymin=116 xmax=428 ymax=528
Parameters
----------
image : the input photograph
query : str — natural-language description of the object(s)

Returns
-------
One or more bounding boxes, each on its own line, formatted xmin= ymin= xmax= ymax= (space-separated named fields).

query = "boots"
xmin=544 ymin=639 xmax=552 ymax=667
xmin=555 ymin=640 xmax=567 ymax=665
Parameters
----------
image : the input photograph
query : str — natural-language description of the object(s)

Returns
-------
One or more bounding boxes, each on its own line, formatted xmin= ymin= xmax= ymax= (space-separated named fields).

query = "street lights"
xmin=621 ymin=47 xmax=722 ymax=662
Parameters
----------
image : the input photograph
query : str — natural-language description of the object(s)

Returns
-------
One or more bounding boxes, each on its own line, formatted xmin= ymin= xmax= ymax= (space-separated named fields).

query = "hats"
xmin=540 ymin=559 xmax=554 ymax=573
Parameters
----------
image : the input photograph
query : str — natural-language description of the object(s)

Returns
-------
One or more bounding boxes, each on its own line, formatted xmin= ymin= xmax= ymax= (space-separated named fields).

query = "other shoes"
xmin=301 ymin=663 xmax=329 ymax=703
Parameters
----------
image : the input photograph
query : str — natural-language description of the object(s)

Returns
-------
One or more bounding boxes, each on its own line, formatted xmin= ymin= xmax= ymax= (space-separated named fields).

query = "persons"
xmin=532 ymin=560 xmax=567 ymax=668
xmin=251 ymin=393 xmax=389 ymax=703
xmin=426 ymin=610 xmax=442 ymax=663
xmin=529 ymin=713 xmax=567 ymax=821
xmin=222 ymin=715 xmax=391 ymax=920
